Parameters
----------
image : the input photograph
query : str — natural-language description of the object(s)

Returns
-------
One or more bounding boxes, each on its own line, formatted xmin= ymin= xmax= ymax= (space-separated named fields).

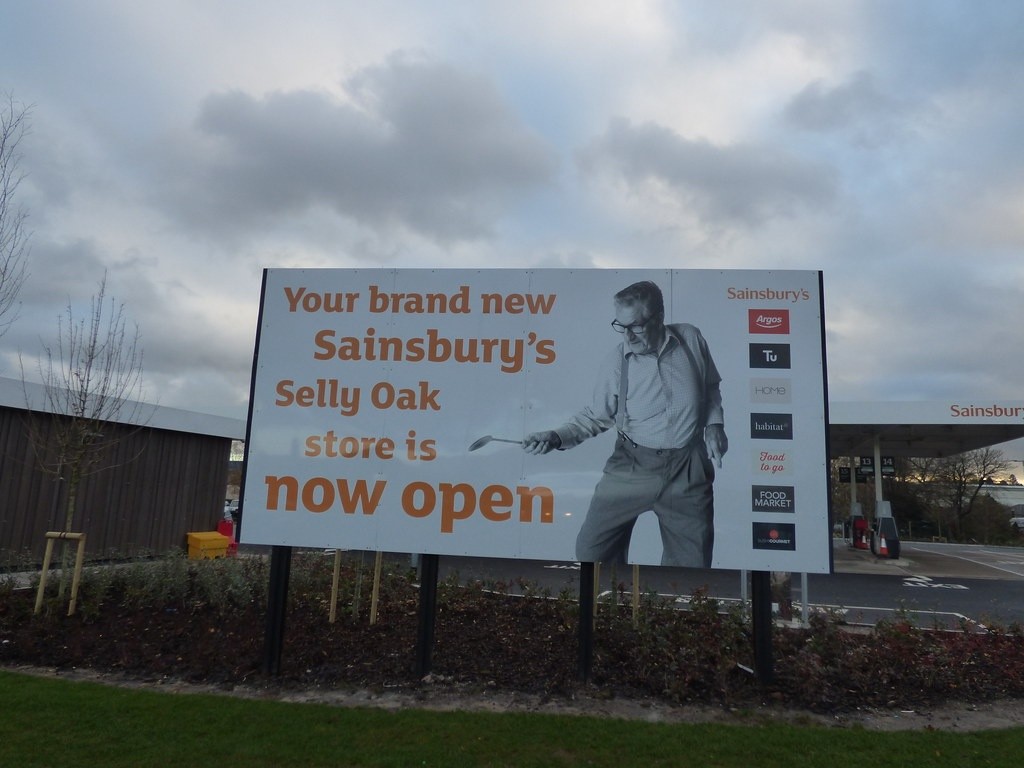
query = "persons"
xmin=523 ymin=281 xmax=728 ymax=569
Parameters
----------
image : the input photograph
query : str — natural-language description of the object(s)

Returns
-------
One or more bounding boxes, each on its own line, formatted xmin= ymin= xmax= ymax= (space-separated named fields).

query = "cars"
xmin=224 ymin=500 xmax=239 ymax=515
xmin=1009 ymin=516 xmax=1024 ymax=530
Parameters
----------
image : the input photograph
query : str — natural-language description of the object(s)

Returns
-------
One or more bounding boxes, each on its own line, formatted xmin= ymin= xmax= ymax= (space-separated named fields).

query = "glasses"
xmin=611 ymin=313 xmax=655 ymax=334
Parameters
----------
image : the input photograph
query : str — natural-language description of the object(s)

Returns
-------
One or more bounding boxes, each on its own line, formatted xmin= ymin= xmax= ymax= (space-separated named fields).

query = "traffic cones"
xmin=880 ymin=533 xmax=889 ymax=554
xmin=860 ymin=531 xmax=868 ymax=548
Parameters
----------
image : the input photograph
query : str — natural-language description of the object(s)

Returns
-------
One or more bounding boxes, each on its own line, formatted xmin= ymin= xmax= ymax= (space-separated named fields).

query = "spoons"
xmin=468 ymin=436 xmax=523 ymax=451
